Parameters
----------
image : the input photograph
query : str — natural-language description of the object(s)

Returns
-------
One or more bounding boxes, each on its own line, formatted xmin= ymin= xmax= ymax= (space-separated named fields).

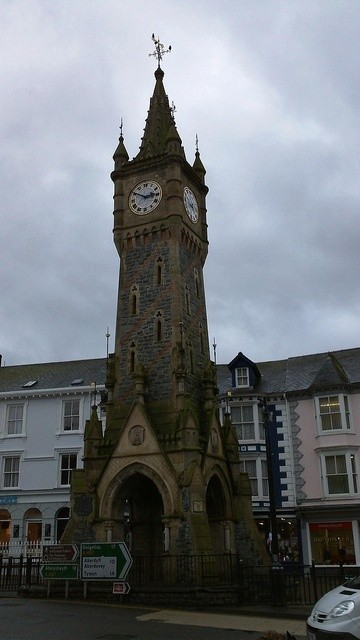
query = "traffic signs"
xmin=41 ymin=544 xmax=79 ymax=562
xmin=113 ymin=582 xmax=130 ymax=594
xmin=80 ymin=542 xmax=133 ymax=581
xmin=39 ymin=564 xmax=78 ymax=579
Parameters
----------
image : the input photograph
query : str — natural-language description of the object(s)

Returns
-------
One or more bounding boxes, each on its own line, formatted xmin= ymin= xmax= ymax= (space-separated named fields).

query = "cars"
xmin=307 ymin=576 xmax=360 ymax=640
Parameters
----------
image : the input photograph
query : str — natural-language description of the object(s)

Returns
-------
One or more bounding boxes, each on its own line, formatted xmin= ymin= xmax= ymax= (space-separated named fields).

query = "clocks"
xmin=128 ymin=180 xmax=162 ymax=215
xmin=183 ymin=186 xmax=199 ymax=224
